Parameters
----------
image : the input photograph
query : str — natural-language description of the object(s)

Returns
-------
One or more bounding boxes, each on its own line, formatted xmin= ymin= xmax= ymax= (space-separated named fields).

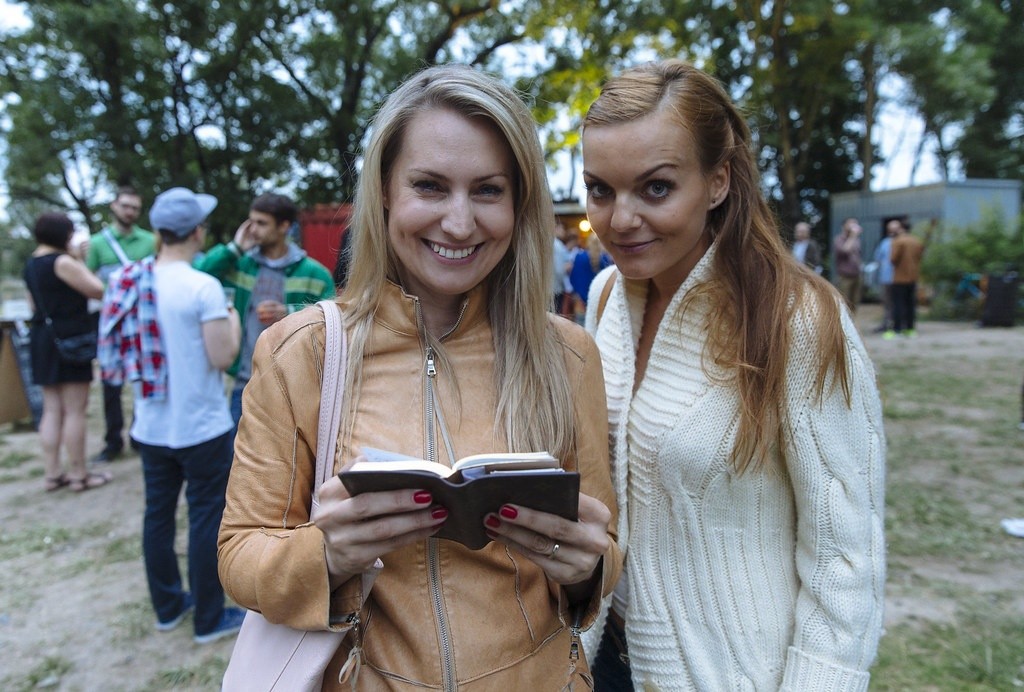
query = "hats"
xmin=148 ymin=186 xmax=219 ymax=238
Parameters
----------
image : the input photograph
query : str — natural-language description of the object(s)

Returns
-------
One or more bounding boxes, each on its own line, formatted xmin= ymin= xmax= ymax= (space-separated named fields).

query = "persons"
xmin=85 ymin=186 xmax=155 ymax=462
xmin=874 ymin=220 xmax=923 ymax=340
xmin=216 ymin=65 xmax=593 ymax=692
xmin=833 ymin=216 xmax=863 ymax=313
xmin=129 ymin=187 xmax=246 ymax=643
xmin=552 ymin=222 xmax=610 ymax=316
xmin=195 ymin=192 xmax=337 ymax=460
xmin=577 ymin=59 xmax=885 ymax=692
xmin=789 ymin=222 xmax=819 ymax=271
xmin=20 ymin=210 xmax=114 ymax=492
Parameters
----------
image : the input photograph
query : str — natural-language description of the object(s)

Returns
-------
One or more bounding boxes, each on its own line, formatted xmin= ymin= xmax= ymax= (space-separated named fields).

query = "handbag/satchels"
xmin=221 ymin=491 xmax=383 ymax=692
xmin=56 ymin=333 xmax=98 ymax=363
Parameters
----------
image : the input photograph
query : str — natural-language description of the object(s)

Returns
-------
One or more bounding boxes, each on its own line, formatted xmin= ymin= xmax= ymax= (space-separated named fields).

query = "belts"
xmin=603 ymin=622 xmax=631 ymax=671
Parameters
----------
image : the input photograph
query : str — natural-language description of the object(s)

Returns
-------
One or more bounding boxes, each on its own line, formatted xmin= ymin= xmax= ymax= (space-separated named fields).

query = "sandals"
xmin=69 ymin=470 xmax=114 ymax=492
xmin=42 ymin=469 xmax=74 ymax=491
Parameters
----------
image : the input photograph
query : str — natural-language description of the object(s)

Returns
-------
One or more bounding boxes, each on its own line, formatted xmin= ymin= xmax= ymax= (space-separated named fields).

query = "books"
xmin=339 ymin=446 xmax=581 ymax=551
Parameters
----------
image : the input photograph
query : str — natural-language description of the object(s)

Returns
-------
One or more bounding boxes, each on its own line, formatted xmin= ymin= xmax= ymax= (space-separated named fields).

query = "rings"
xmin=548 ymin=540 xmax=560 ymax=560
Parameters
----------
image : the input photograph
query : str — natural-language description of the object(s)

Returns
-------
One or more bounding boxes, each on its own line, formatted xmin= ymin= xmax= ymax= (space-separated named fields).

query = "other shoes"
xmin=194 ymin=607 xmax=247 ymax=644
xmin=154 ymin=592 xmax=193 ymax=631
xmin=1000 ymin=517 xmax=1024 ymax=537
xmin=881 ymin=330 xmax=901 ymax=340
xmin=899 ymin=329 xmax=918 ymax=338
xmin=96 ymin=441 xmax=122 ymax=461
